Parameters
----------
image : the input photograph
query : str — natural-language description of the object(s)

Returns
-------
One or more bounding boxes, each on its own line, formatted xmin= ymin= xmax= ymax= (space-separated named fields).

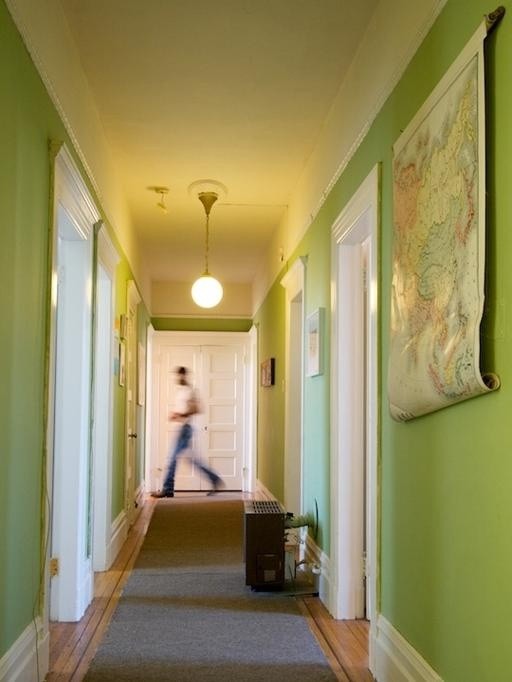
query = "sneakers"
xmin=151 ymin=491 xmax=174 ymax=498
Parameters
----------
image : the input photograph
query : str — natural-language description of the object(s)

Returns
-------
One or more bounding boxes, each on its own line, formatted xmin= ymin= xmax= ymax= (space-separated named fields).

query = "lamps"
xmin=188 ymin=191 xmax=226 ymax=310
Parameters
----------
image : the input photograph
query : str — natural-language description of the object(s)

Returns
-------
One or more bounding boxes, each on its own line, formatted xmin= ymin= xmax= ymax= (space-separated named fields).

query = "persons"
xmin=149 ymin=366 xmax=226 ymax=498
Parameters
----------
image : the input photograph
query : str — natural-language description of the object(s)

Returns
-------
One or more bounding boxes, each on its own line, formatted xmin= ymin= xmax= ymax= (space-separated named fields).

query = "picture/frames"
xmin=306 ymin=306 xmax=325 ymax=379
